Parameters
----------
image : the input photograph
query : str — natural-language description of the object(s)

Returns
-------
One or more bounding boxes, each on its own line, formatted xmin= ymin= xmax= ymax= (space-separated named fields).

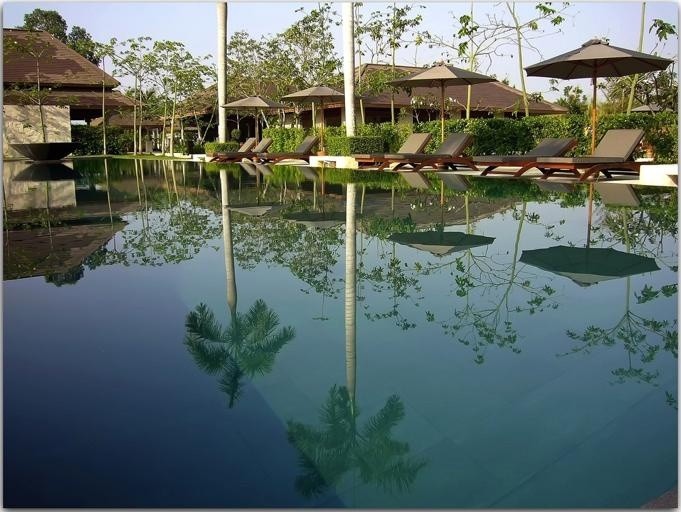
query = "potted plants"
xmin=12 ymin=164 xmax=87 ymax=288
xmin=3 ymin=26 xmax=82 ymax=163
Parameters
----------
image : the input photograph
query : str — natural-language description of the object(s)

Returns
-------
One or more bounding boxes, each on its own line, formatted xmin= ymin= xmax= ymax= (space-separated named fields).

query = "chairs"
xmin=386 ymin=132 xmax=480 ymax=172
xmin=538 ymin=129 xmax=647 ymax=178
xmin=349 ymin=132 xmax=437 ymax=172
xmin=483 ymin=138 xmax=582 ymax=178
xmin=257 ymin=136 xmax=321 ymax=164
xmin=212 ymin=134 xmax=272 ymax=163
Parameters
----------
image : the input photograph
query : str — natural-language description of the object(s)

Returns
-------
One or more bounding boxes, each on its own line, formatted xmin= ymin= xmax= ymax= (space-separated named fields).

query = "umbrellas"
xmin=522 ymin=39 xmax=674 ymax=156
xmin=281 ymin=168 xmax=362 ymax=229
xmin=518 ymin=183 xmax=662 ymax=288
xmin=228 ymin=168 xmax=289 ymax=217
xmin=280 ymin=85 xmax=345 ymax=152
xmin=220 ymin=96 xmax=290 ymax=147
xmin=383 ymin=63 xmax=500 ymax=143
xmin=384 ymin=181 xmax=497 ymax=259
